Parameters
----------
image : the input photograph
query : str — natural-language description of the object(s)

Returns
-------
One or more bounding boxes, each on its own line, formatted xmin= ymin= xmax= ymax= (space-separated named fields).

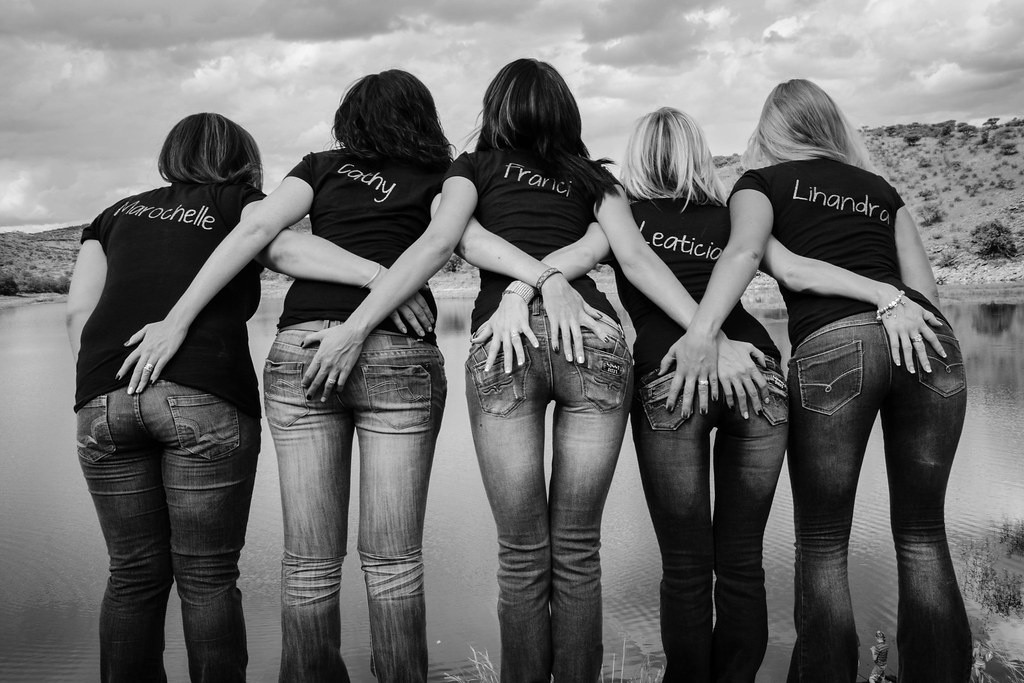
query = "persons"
xmin=299 ymin=58 xmax=771 ymax=683
xmin=113 ymin=68 xmax=609 ymax=683
xmin=656 ymin=77 xmax=974 ymax=683
xmin=470 ymin=102 xmax=948 ymax=683
xmin=65 ymin=111 xmax=436 ymax=683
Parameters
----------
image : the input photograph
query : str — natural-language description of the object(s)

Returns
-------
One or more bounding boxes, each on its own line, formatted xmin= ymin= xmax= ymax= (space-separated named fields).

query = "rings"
xmin=911 ymin=334 xmax=923 ymax=343
xmin=698 ymin=380 xmax=709 ymax=385
xmin=145 ymin=363 xmax=154 ymax=371
xmin=512 ymin=330 xmax=518 ymax=336
xmin=330 ymin=380 xmax=336 ymax=383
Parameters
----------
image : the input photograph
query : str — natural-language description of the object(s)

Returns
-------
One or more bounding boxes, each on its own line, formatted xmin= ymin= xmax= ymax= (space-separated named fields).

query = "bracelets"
xmin=876 ymin=290 xmax=906 ymax=320
xmin=361 ymin=264 xmax=381 ymax=288
xmin=502 ymin=280 xmax=535 ymax=303
xmin=537 ymin=268 xmax=563 ymax=294
